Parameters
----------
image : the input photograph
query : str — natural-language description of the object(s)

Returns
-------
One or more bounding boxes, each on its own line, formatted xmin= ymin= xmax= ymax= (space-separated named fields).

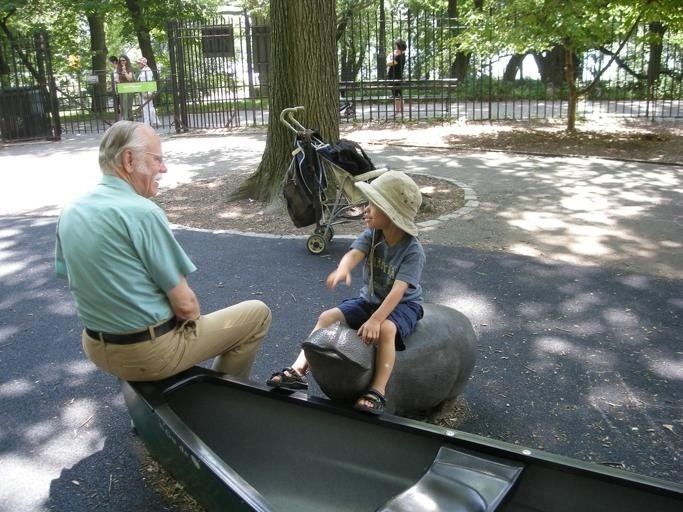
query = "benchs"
xmin=330 ymin=77 xmax=459 ymax=120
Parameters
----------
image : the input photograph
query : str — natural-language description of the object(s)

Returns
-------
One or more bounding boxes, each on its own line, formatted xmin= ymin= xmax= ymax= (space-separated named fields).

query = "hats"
xmin=135 ymin=57 xmax=149 ymax=66
xmin=354 ymin=169 xmax=422 ymax=237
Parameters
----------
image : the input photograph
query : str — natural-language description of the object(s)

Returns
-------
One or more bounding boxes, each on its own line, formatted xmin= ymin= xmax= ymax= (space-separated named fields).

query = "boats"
xmin=119 ymin=365 xmax=683 ymax=511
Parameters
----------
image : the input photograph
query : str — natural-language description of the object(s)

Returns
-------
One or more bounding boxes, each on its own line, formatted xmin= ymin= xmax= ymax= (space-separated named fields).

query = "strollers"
xmin=276 ymin=104 xmax=390 ymax=257
xmin=340 ymin=102 xmax=356 ymax=117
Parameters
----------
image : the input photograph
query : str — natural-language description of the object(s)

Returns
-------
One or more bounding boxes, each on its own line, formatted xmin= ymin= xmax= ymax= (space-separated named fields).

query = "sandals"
xmin=265 ymin=368 xmax=309 ymax=389
xmin=353 ymin=388 xmax=387 ymax=415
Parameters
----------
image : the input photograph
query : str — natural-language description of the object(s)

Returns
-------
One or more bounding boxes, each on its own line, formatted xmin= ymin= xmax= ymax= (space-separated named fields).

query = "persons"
xmin=116 ymin=54 xmax=134 ymax=121
xmin=135 ymin=57 xmax=161 ymax=126
xmin=108 ymin=56 xmax=119 ymax=119
xmin=386 ymin=50 xmax=400 ymax=75
xmin=386 ymin=40 xmax=406 ymax=116
xmin=265 ymin=169 xmax=422 ymax=416
xmin=54 ymin=121 xmax=272 ymax=384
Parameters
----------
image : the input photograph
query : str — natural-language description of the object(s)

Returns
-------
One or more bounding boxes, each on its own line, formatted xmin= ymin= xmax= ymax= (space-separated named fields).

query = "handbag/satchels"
xmin=280 ymin=157 xmax=326 ymax=228
xmin=334 ymin=138 xmax=374 ymax=176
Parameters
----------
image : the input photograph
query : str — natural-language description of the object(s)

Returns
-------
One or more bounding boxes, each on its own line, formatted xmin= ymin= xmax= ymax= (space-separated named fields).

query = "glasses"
xmin=144 ymin=151 xmax=166 ymax=163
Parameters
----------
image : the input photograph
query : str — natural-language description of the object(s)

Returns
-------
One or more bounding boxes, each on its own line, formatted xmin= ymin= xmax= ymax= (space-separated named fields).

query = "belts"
xmin=85 ymin=316 xmax=178 ymax=345
